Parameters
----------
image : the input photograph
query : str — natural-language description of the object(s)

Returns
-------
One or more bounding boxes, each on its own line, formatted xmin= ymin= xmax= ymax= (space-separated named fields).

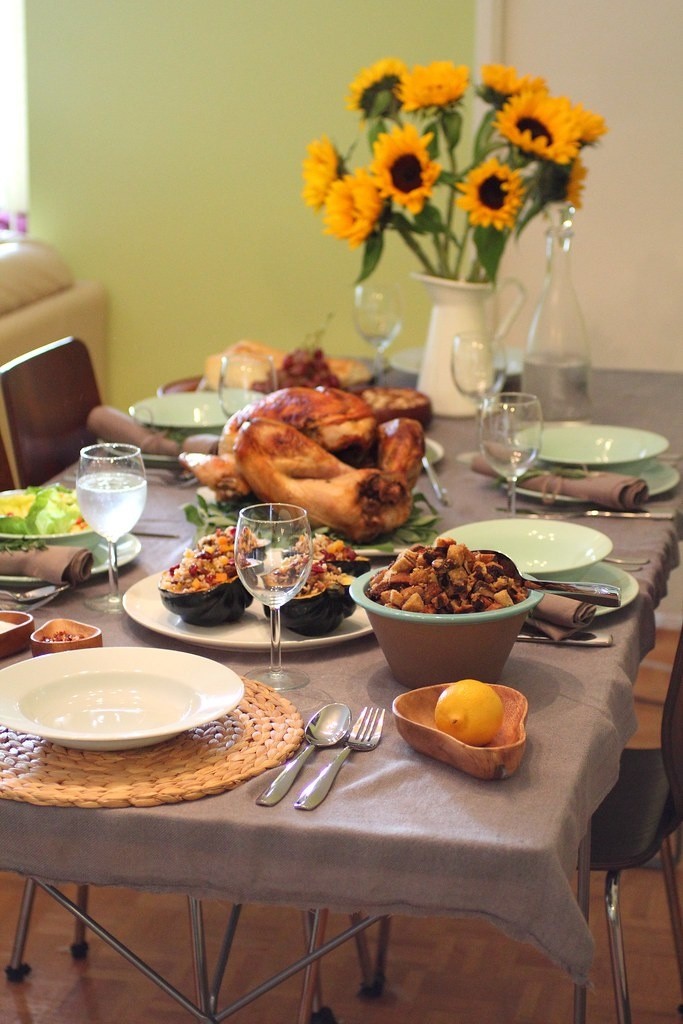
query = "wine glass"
xmin=476 ymin=391 xmax=544 ymax=519
xmin=447 ymin=332 xmax=520 ymax=465
xmin=231 ymin=503 xmax=311 ymax=693
xmin=76 ymin=442 xmax=148 ymax=617
xmin=350 ymin=281 xmax=403 ymax=393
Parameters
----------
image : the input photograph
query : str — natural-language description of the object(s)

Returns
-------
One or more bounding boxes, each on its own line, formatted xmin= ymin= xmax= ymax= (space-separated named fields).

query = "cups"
xmin=220 ymin=353 xmax=278 ymax=420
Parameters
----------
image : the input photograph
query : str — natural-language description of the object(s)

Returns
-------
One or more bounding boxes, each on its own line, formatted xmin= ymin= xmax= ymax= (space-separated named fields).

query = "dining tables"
xmin=0 ymin=353 xmax=683 ymax=1023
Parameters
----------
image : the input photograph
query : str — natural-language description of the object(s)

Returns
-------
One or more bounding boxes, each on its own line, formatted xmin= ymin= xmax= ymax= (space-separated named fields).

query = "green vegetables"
xmin=1 ymin=485 xmax=85 ymax=536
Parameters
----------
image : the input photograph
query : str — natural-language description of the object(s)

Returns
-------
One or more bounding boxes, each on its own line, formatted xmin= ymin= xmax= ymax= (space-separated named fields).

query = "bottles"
xmin=519 ymin=188 xmax=595 ymax=425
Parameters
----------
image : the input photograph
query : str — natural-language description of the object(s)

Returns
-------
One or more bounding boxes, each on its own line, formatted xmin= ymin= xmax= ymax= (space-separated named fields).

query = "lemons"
xmin=435 ymin=678 xmax=503 ymax=746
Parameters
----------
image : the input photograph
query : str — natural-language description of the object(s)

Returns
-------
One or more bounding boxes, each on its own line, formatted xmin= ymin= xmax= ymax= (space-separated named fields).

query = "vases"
xmin=409 ymin=270 xmax=506 ymax=418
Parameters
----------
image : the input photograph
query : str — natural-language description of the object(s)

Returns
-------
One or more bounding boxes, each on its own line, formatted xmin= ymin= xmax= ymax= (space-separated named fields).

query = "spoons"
xmin=469 ymin=547 xmax=624 ymax=609
xmin=0 ymin=584 xmax=71 ymax=603
xmin=253 ymin=703 xmax=353 ymax=806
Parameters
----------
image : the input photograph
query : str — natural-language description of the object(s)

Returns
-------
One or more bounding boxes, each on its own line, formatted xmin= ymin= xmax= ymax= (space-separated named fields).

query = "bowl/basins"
xmin=128 ymin=390 xmax=267 ymax=445
xmin=0 ymin=487 xmax=108 ymax=540
xmin=348 ymin=562 xmax=545 ymax=689
xmin=432 ymin=518 xmax=614 ymax=586
xmin=514 ymin=426 xmax=672 ymax=481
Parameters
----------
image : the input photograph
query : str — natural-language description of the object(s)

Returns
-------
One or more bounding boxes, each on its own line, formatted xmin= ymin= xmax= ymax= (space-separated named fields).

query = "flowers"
xmin=299 ymin=58 xmax=612 ymax=285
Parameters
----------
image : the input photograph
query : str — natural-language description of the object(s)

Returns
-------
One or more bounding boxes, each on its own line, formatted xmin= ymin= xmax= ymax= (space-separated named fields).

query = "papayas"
xmin=159 ymin=530 xmax=371 ymax=637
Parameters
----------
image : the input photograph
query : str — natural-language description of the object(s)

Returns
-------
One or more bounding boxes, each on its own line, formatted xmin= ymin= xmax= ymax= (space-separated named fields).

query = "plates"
xmin=0 ymin=647 xmax=246 ymax=750
xmin=494 ymin=461 xmax=680 ymax=505
xmin=122 ymin=566 xmax=380 ymax=651
xmin=511 ymin=560 xmax=640 ymax=639
xmin=0 ymin=532 xmax=146 ymax=583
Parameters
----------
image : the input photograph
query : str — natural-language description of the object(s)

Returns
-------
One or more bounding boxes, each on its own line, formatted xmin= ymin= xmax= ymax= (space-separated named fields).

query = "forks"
xmin=293 ymin=707 xmax=387 ymax=809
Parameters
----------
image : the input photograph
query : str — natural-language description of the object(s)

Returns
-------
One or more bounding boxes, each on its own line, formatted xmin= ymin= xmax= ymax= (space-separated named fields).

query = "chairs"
xmin=0 ymin=335 xmax=102 ymax=490
xmin=296 ymin=621 xmax=683 ymax=1023
